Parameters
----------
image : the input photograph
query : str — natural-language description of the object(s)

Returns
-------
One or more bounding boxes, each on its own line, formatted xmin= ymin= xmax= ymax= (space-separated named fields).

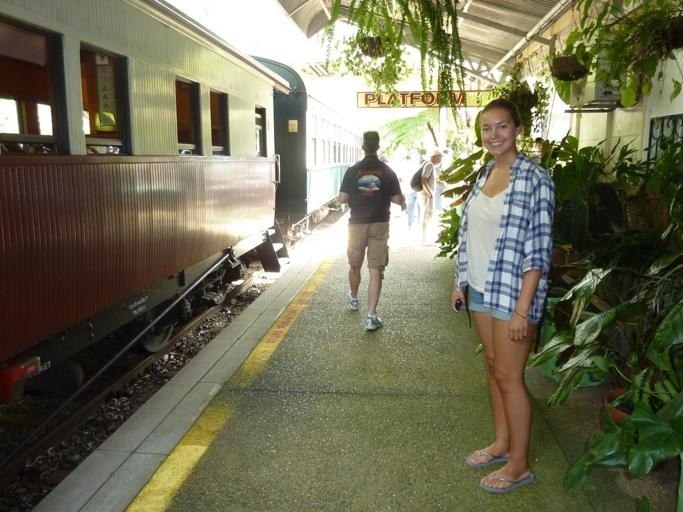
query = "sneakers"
xmin=348 ymin=295 xmax=358 ymax=310
xmin=367 ymin=317 xmax=381 ymax=330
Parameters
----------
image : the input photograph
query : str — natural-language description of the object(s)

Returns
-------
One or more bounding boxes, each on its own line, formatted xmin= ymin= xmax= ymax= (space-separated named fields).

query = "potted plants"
xmin=523 ymin=1 xmax=683 ymax=495
xmin=318 ymin=1 xmax=471 ymax=129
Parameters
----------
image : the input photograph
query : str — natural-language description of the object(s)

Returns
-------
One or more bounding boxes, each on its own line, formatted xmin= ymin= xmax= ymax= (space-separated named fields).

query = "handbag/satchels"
xmin=411 ymin=161 xmax=432 ymax=191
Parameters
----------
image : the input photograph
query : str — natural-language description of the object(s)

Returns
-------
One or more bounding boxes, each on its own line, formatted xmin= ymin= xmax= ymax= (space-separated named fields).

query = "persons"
xmin=451 ymin=98 xmax=556 ymax=494
xmin=338 ymin=131 xmax=403 ymax=330
xmin=397 ymin=148 xmax=450 ymax=231
xmin=530 ymin=137 xmax=544 ymax=164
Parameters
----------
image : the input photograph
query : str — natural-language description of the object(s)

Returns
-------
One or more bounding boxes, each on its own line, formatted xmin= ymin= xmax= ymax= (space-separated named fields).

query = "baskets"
xmin=623 ymin=193 xmax=668 ymax=230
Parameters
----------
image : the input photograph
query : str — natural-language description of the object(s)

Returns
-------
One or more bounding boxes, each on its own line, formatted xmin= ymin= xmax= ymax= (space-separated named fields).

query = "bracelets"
xmin=514 ymin=311 xmax=528 ymax=320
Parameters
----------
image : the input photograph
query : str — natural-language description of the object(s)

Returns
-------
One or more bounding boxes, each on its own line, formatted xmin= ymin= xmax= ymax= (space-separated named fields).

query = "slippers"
xmin=466 ymin=450 xmax=534 ymax=493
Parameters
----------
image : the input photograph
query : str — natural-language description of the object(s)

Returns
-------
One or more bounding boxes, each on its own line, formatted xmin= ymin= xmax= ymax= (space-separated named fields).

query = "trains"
xmin=0 ymin=1 xmax=378 ymax=422
